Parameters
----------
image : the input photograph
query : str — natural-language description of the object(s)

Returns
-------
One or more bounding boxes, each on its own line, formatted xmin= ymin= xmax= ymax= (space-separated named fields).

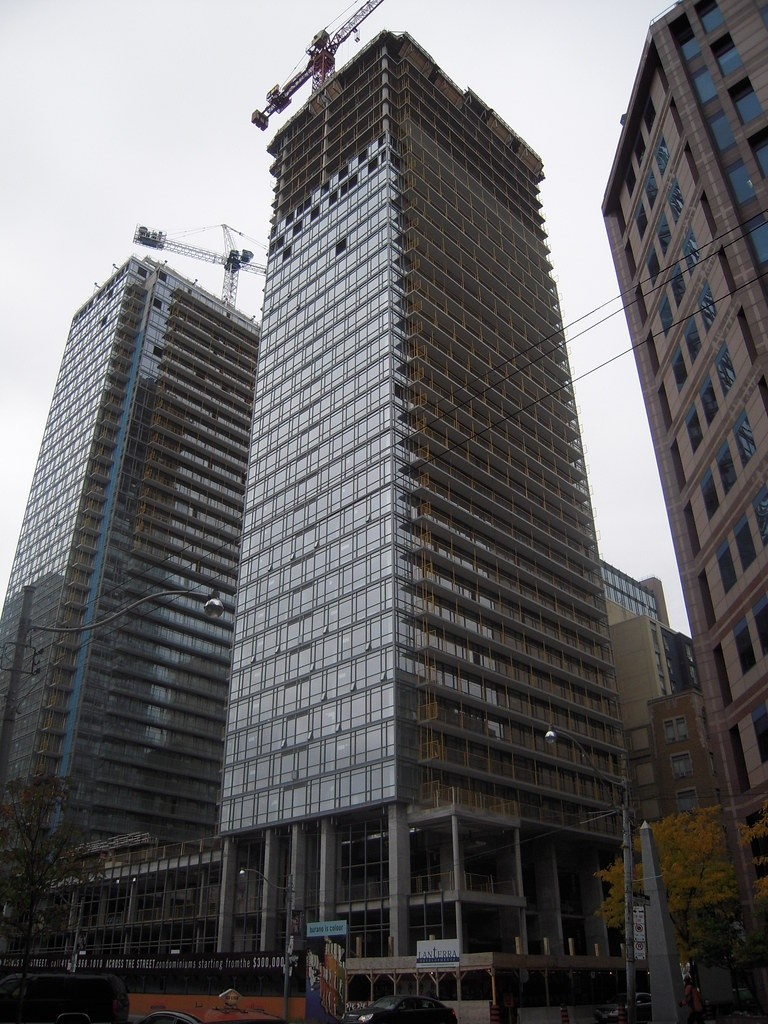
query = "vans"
xmin=0 ymin=972 xmax=129 ymax=1024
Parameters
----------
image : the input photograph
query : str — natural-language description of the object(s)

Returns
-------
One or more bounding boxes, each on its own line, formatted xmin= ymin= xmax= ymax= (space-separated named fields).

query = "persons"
xmin=678 ymin=976 xmax=706 ymax=1024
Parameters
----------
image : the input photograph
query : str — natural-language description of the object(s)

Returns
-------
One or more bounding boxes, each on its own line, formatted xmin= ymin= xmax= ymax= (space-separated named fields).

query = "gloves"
xmin=679 ymin=1002 xmax=683 ymax=1007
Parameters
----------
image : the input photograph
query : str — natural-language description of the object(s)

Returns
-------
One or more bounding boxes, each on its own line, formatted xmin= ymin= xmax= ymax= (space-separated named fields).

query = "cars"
xmin=593 ymin=991 xmax=652 ymax=1024
xmin=699 ymin=987 xmax=761 ymax=1020
xmin=338 ymin=994 xmax=457 ymax=1024
xmin=133 ymin=988 xmax=290 ymax=1024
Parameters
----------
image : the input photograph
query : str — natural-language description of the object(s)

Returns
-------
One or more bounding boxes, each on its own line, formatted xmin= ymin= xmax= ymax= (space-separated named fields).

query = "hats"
xmin=684 ymin=976 xmax=690 ymax=982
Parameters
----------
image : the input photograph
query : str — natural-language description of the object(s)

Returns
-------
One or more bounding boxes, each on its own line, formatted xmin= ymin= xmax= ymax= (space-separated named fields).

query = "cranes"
xmin=251 ymin=0 xmax=383 ymax=132
xmin=133 ymin=223 xmax=269 ymax=309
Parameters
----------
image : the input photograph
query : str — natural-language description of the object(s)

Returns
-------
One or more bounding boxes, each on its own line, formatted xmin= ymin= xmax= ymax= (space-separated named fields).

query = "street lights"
xmin=543 ymin=722 xmax=638 ymax=1024
xmin=239 ymin=868 xmax=293 ymax=1024
xmin=0 ymin=585 xmax=225 ymax=796
